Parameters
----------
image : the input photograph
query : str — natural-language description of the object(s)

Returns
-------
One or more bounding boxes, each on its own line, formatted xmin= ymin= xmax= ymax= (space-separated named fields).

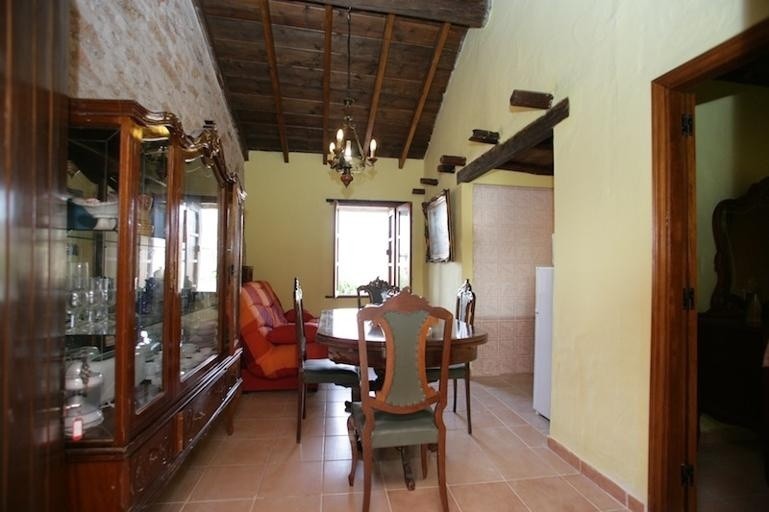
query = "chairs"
xmin=425 ymin=279 xmax=475 ymax=433
xmin=294 ymin=277 xmax=362 ymax=444
xmin=347 ymin=287 xmax=453 ymax=512
xmin=357 ymin=277 xmax=400 ymax=391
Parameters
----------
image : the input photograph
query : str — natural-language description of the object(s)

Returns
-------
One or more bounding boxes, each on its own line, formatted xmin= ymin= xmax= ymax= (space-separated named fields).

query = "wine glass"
xmin=65 ymin=261 xmax=116 ymax=334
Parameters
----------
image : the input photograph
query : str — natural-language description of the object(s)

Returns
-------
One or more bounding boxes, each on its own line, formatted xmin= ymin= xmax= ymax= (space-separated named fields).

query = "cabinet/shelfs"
xmin=698 ymin=315 xmax=769 ymax=436
xmin=62 ymin=96 xmax=248 ymax=512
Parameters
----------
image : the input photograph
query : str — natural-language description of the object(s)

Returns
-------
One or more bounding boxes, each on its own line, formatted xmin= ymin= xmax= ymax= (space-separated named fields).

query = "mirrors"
xmin=711 ymin=176 xmax=769 ymax=315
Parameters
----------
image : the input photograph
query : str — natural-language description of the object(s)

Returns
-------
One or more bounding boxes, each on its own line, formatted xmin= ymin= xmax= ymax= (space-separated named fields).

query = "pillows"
xmin=266 ymin=322 xmax=318 ymax=344
xmin=284 ymin=308 xmax=315 ymax=323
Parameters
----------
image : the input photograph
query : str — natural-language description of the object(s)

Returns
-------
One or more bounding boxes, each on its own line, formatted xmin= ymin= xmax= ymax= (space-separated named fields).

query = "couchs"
xmin=239 ymin=280 xmax=328 ymax=393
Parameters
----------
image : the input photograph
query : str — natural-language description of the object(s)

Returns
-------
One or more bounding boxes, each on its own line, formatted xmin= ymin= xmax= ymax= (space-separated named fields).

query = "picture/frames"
xmin=423 ymin=189 xmax=453 ymax=263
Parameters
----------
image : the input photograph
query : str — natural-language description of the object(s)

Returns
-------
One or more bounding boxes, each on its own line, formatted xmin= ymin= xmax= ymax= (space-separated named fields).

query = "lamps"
xmin=326 ymin=5 xmax=377 ymax=185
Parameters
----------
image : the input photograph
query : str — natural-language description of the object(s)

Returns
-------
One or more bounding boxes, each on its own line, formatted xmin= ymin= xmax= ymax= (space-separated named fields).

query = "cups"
xmin=183 ymin=343 xmax=199 ymax=354
